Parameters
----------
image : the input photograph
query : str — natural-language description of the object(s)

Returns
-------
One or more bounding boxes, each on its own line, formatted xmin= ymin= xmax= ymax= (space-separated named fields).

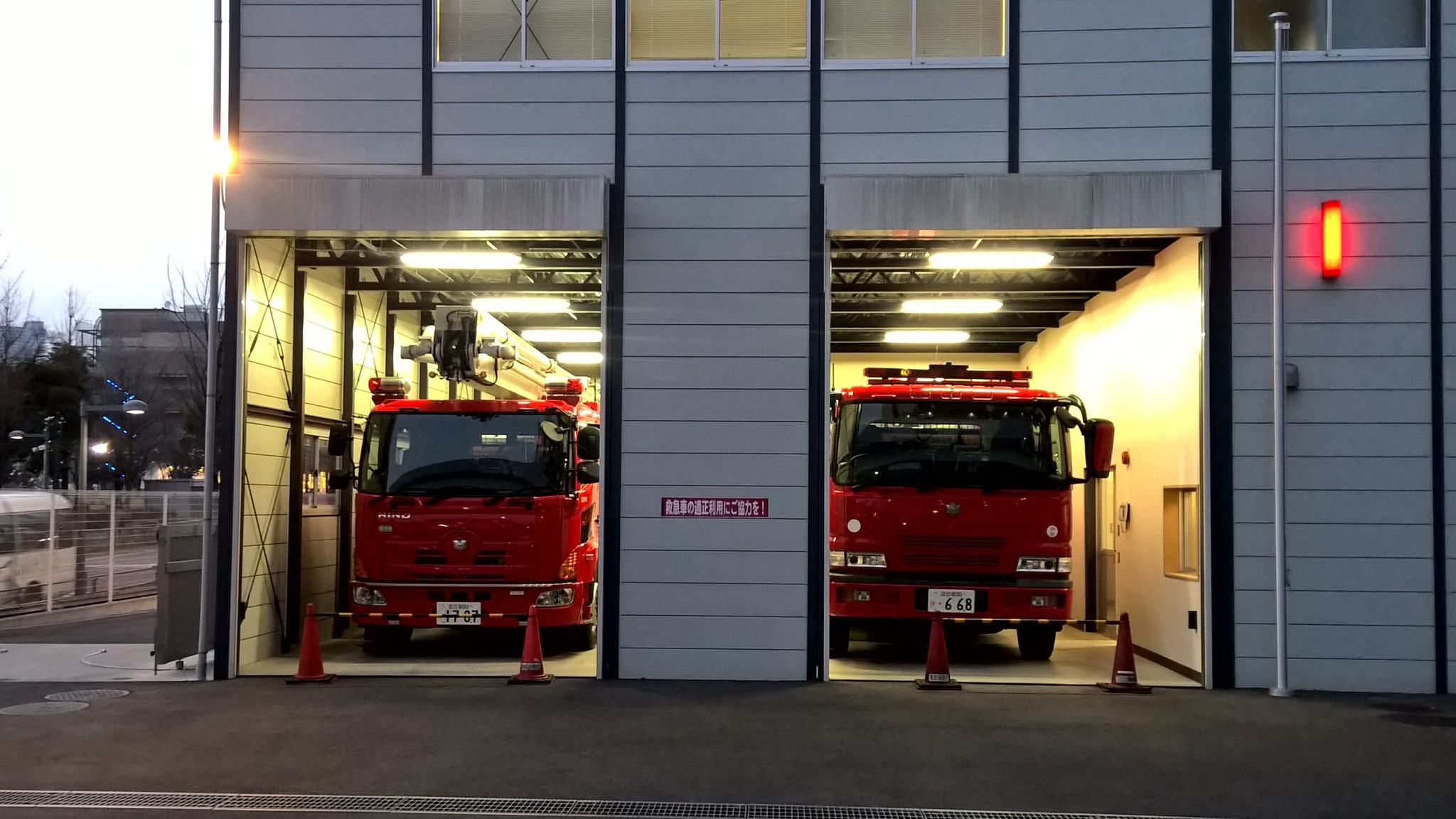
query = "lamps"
xmin=1320 ymin=200 xmax=1343 ymax=280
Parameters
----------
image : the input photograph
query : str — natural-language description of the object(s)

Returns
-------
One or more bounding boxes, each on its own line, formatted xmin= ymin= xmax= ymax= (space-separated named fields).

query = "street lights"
xmin=75 ymin=400 xmax=149 ymax=594
xmin=8 ymin=425 xmax=50 ymax=488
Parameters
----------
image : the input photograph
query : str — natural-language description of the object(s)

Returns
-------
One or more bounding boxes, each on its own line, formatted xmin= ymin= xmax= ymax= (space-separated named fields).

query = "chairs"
xmin=854 ymin=424 xmax=884 ymax=456
xmin=982 ymin=418 xmax=1037 ymax=468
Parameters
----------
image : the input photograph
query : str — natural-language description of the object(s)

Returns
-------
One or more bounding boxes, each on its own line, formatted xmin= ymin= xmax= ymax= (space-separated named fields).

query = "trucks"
xmin=328 ymin=376 xmax=601 ymax=657
xmin=830 ymin=360 xmax=1115 ymax=661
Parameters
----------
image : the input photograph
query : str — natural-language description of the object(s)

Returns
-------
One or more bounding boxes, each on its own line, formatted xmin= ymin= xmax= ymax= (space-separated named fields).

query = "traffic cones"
xmin=507 ymin=605 xmax=556 ymax=685
xmin=285 ymin=603 xmax=337 ymax=684
xmin=1096 ymin=611 xmax=1153 ymax=695
xmin=913 ymin=611 xmax=963 ymax=691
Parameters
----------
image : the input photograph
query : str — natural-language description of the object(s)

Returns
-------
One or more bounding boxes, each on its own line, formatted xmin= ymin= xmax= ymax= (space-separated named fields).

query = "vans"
xmin=0 ymin=489 xmax=77 ymax=610
